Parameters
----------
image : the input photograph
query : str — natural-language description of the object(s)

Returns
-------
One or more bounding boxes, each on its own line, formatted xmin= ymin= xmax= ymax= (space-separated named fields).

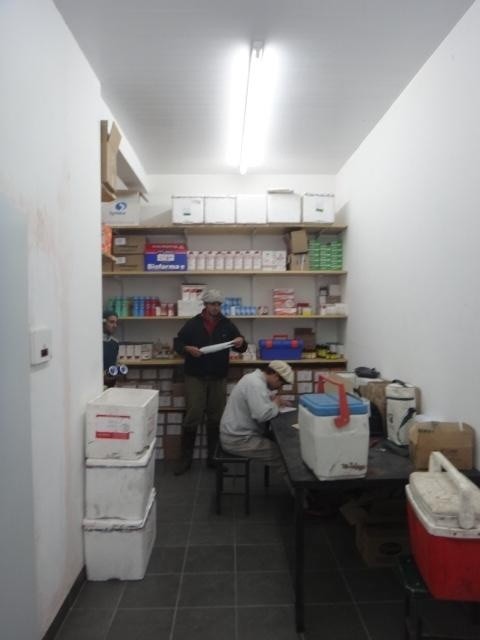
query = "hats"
xmin=201 ymin=290 xmax=224 ymax=304
xmin=269 ymin=360 xmax=295 ymax=384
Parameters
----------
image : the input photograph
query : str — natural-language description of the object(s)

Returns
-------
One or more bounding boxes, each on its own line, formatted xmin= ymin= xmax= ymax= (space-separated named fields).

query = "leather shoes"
xmin=172 ymin=457 xmax=192 ymax=474
xmin=207 ymin=459 xmax=228 ymax=471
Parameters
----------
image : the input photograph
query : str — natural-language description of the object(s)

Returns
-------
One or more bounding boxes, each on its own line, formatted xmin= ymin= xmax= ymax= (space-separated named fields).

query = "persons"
xmin=169 ymin=289 xmax=247 ymax=476
xmin=220 ymin=359 xmax=322 ymax=516
xmin=103 ymin=313 xmax=120 ymax=386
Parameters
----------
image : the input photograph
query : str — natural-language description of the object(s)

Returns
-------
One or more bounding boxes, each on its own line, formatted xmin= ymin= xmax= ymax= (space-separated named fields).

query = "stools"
xmin=213 ymin=439 xmax=252 ymax=516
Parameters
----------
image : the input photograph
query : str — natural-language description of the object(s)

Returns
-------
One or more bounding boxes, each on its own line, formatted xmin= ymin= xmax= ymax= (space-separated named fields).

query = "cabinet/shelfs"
xmin=102 ymin=222 xmax=348 ymax=465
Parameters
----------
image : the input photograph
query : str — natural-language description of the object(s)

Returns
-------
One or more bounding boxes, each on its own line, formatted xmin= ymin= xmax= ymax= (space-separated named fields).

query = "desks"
xmin=264 ymin=399 xmax=479 ymax=634
xmin=392 ymin=544 xmax=480 ymax=639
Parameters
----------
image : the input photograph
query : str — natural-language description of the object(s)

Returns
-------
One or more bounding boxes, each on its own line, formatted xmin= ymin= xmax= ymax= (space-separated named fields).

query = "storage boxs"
xmin=258 ymin=334 xmax=303 ymax=361
xmin=102 ymin=230 xmax=344 ymax=273
xmin=81 ymin=385 xmax=160 ymax=583
xmin=101 ymin=189 xmax=150 ymax=227
xmin=116 ymin=364 xmax=346 ymax=465
xmin=403 ymin=420 xmax=479 ymax=601
xmin=171 ymin=193 xmax=335 ymax=227
xmin=297 ymin=376 xmax=371 ymax=482
